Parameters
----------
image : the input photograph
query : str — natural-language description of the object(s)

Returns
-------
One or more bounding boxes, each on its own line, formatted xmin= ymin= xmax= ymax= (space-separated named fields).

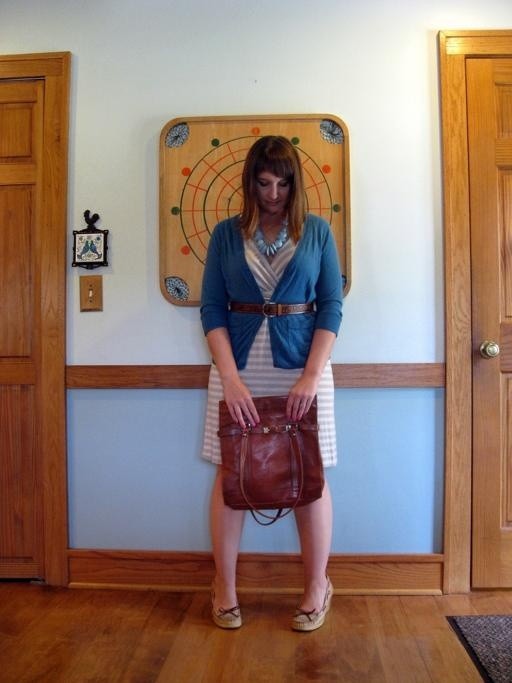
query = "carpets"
xmin=445 ymin=614 xmax=512 ymax=682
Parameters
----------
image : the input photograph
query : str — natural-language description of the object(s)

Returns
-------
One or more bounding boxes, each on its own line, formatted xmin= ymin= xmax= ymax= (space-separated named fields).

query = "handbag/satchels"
xmin=217 ymin=394 xmax=324 ymax=510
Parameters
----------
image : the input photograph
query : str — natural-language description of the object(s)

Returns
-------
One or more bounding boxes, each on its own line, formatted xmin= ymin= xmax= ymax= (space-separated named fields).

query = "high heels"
xmin=290 ymin=573 xmax=335 ymax=632
xmin=210 ymin=581 xmax=242 ymax=630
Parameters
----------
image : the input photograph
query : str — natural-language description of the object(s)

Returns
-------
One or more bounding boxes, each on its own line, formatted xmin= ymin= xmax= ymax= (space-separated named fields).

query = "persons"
xmin=200 ymin=135 xmax=343 ymax=632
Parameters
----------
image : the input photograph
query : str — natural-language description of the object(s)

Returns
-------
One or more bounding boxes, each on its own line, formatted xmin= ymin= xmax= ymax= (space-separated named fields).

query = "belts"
xmin=227 ymin=301 xmax=313 ymax=317
